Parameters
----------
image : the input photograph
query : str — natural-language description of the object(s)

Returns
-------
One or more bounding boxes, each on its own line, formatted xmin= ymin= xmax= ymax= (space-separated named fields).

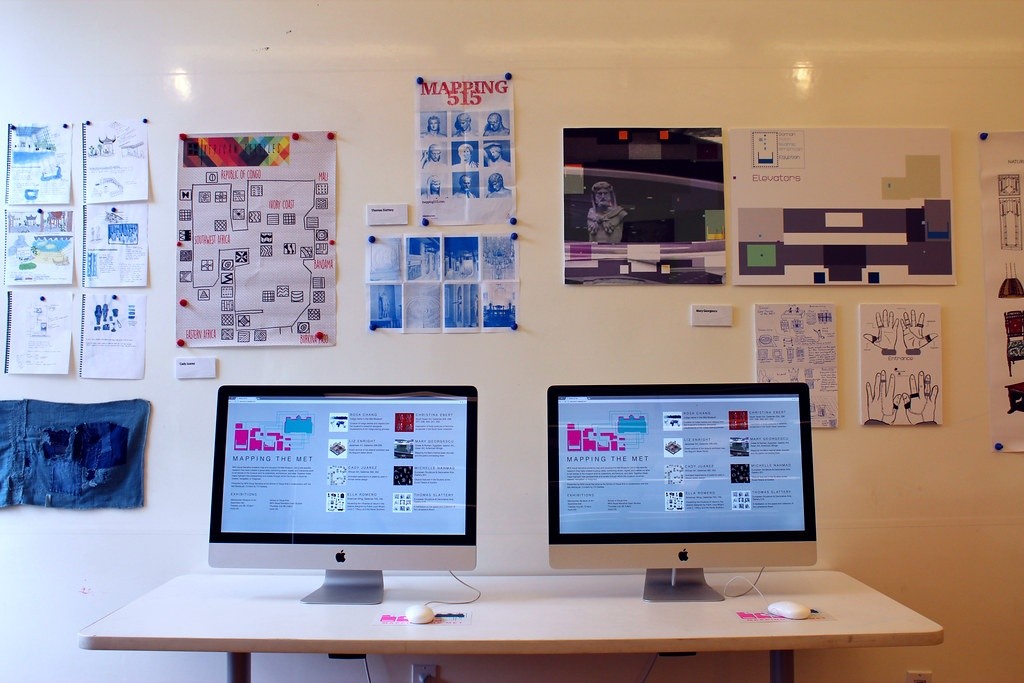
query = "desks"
xmin=80 ymin=573 xmax=944 ymax=683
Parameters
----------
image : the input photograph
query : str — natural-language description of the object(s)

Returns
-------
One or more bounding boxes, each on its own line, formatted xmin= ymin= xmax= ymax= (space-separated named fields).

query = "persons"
xmin=587 ymin=181 xmax=626 ymax=244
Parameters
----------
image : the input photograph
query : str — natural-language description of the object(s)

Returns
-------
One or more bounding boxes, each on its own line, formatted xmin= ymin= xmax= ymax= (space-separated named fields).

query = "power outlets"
xmin=412 ymin=664 xmax=437 ymax=683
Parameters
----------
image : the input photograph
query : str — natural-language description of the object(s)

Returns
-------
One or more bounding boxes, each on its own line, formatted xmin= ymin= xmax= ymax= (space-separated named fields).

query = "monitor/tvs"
xmin=207 ymin=383 xmax=479 ymax=605
xmin=546 ymin=384 xmax=818 ymax=601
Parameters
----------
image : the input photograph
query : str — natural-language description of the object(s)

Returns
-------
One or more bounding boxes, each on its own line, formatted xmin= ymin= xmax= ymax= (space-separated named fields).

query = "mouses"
xmin=405 ymin=606 xmax=435 ymax=623
xmin=768 ymin=600 xmax=811 ymax=620
